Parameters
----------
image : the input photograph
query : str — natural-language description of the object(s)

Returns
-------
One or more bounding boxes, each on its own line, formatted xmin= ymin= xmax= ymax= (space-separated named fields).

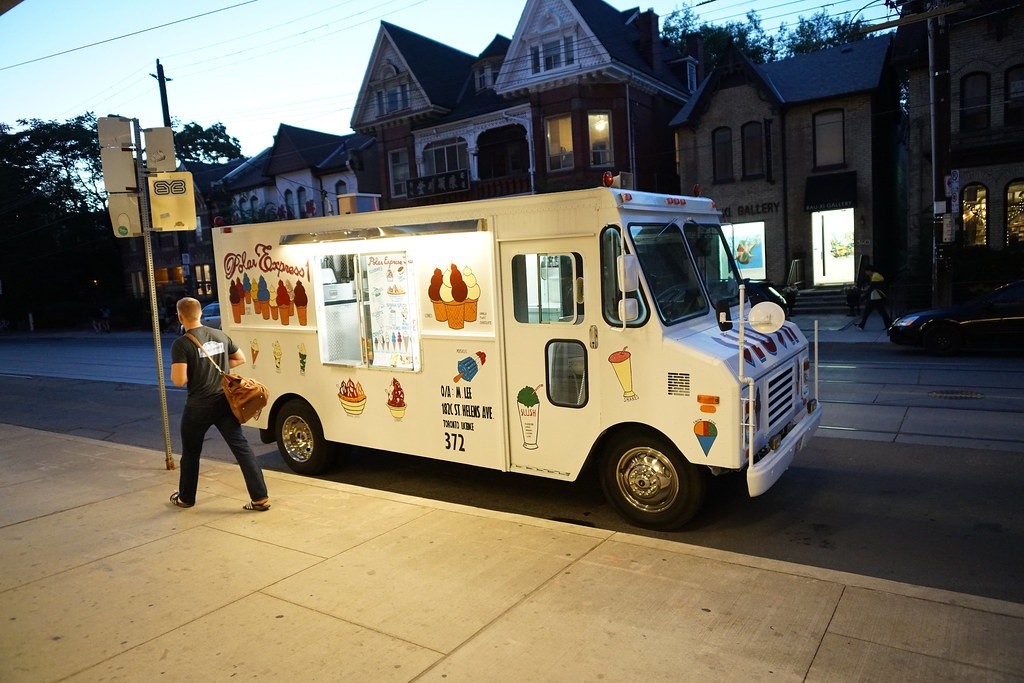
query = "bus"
xmin=210 ymin=186 xmax=822 ymax=528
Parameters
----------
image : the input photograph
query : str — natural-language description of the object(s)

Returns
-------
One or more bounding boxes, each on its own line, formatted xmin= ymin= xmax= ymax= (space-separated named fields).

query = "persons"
xmin=853 ymin=265 xmax=892 ymax=331
xmin=101 ymin=306 xmax=112 ymax=333
xmin=153 ymin=301 xmax=167 ymax=335
xmin=92 ymin=308 xmax=103 ymax=334
xmin=169 ymin=297 xmax=271 ymax=512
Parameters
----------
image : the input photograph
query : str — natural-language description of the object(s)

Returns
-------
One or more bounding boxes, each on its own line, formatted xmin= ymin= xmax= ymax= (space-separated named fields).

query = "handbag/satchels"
xmin=870 ymin=287 xmax=887 ymax=300
xmin=222 ymin=373 xmax=267 ymax=424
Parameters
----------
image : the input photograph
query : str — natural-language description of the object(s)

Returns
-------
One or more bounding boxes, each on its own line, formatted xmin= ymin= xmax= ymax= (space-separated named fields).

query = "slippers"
xmin=244 ymin=501 xmax=269 ymax=511
xmin=169 ymin=492 xmax=195 ymax=507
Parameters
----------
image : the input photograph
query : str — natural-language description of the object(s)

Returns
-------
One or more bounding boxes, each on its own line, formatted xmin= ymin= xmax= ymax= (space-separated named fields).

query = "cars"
xmin=887 ymin=279 xmax=1024 ymax=357
xmin=180 ymin=302 xmax=223 ymax=335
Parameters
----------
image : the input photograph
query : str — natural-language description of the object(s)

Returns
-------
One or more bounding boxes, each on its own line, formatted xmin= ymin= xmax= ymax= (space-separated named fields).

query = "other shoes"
xmin=853 ymin=322 xmax=864 ymax=331
